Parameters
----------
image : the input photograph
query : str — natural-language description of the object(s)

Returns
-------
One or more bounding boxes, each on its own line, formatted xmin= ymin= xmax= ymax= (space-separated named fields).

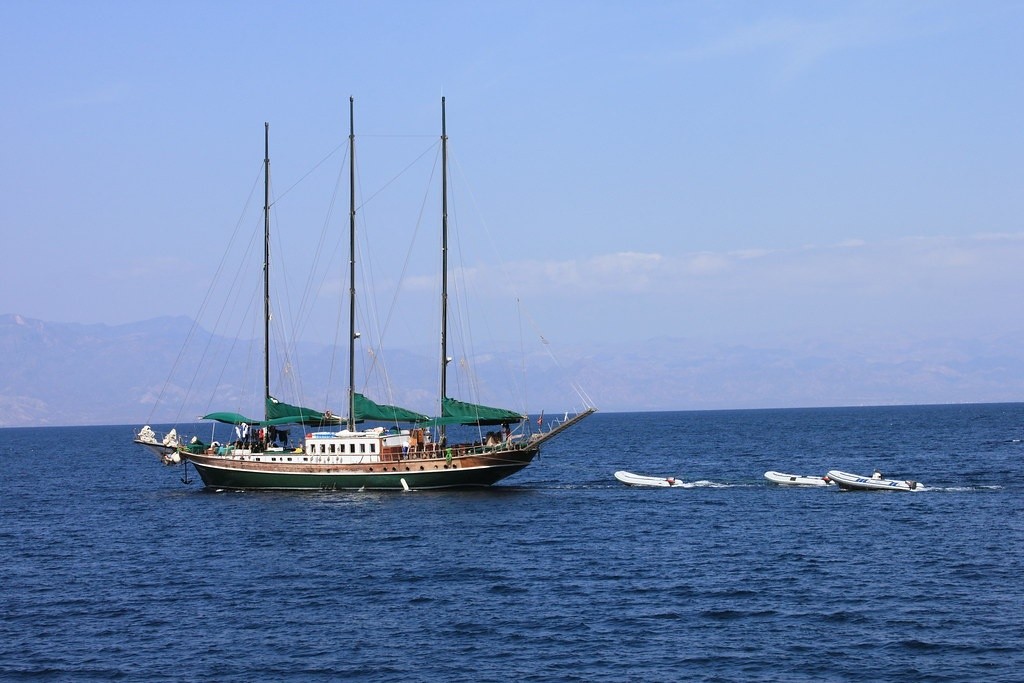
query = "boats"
xmin=764 ymin=469 xmax=836 ymax=486
xmin=827 ymin=470 xmax=925 ymax=493
xmin=614 ymin=470 xmax=682 ymax=487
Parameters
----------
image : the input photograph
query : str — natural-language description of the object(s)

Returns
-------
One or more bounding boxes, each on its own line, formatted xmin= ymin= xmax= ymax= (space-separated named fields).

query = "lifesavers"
xmin=324 ymin=409 xmax=333 ymax=420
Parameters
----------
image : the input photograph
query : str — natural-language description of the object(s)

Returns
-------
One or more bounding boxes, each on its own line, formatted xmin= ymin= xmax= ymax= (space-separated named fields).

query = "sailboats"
xmin=132 ymin=96 xmax=596 ymax=494
